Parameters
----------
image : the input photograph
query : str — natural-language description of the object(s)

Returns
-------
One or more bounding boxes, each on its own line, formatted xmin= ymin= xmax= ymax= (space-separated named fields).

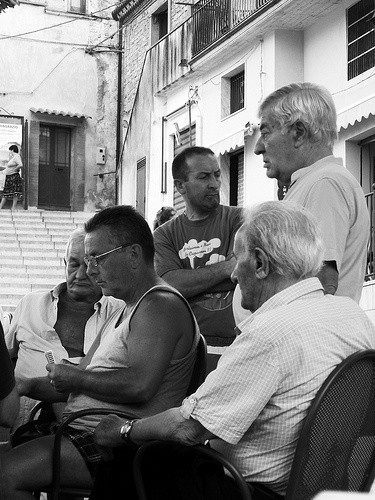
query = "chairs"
xmin=27 ymin=349 xmax=375 ymax=500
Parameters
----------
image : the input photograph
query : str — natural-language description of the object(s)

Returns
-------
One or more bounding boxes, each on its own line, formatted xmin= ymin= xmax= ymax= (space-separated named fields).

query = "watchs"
xmin=119 ymin=419 xmax=141 ymax=446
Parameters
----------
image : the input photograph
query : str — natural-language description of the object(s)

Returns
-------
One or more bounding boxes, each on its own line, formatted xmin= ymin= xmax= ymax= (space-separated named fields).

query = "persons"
xmin=231 ymin=82 xmax=370 ymax=327
xmin=153 ymin=147 xmax=249 ymax=376
xmin=0 ymin=145 xmax=23 ymax=210
xmin=0 ymin=230 xmax=124 ymax=442
xmin=0 ymin=205 xmax=201 ymax=500
xmin=94 ymin=201 xmax=375 ymax=500
xmin=154 ymin=206 xmax=177 ymax=230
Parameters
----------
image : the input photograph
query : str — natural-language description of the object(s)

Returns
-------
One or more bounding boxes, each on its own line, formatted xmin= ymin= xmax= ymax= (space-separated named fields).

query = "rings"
xmin=50 ymin=380 xmax=53 ymax=387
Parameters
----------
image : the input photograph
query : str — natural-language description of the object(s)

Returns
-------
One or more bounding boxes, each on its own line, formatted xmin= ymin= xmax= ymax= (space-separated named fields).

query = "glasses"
xmin=82 ymin=242 xmax=136 ymax=267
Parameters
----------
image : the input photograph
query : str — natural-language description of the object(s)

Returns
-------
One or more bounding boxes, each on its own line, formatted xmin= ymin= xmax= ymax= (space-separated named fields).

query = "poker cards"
xmin=46 ymin=351 xmax=56 ymax=364
